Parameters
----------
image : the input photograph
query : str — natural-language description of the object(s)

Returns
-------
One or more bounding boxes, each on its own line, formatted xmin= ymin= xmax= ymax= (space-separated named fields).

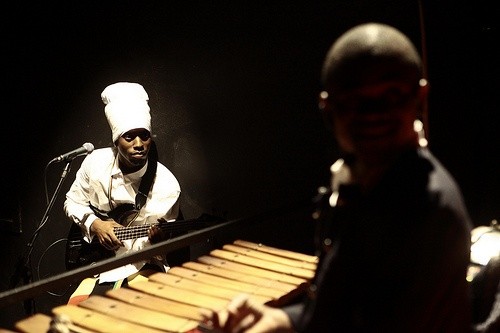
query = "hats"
xmin=101 ymin=82 xmax=151 ymax=147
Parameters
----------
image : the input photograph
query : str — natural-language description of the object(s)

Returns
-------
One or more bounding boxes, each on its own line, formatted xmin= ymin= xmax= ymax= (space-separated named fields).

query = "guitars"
xmin=64 ymin=204 xmax=223 ymax=272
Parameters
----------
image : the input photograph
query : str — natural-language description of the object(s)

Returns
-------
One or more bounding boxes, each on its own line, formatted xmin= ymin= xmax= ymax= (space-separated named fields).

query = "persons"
xmin=197 ymin=23 xmax=471 ymax=333
xmin=62 ymin=82 xmax=182 ymax=292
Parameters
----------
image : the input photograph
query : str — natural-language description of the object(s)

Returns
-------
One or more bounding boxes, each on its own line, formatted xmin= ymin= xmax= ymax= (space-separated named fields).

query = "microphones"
xmin=54 ymin=143 xmax=94 ymax=163
